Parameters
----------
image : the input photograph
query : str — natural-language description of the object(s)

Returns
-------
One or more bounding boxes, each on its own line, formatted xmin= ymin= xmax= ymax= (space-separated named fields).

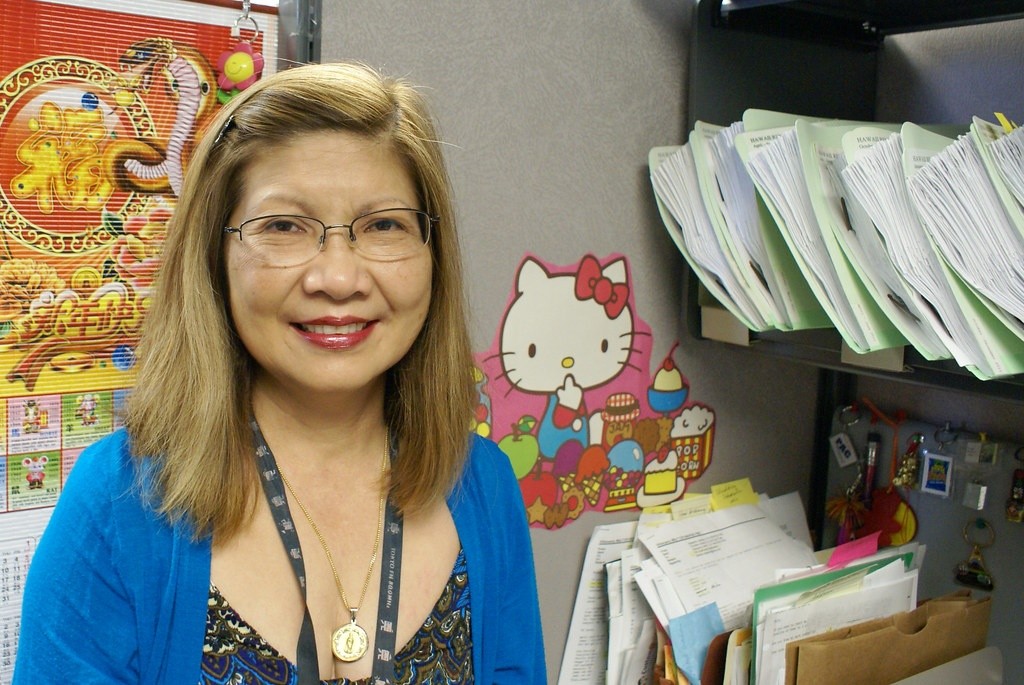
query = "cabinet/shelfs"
xmin=686 ymin=0 xmax=1024 ymax=402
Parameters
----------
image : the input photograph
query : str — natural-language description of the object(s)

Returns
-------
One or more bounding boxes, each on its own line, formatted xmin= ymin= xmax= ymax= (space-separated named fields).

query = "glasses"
xmin=223 ymin=207 xmax=440 ymax=269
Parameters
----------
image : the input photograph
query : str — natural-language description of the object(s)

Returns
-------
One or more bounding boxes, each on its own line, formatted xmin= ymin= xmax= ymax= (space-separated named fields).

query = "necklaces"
xmin=273 ymin=419 xmax=390 ymax=662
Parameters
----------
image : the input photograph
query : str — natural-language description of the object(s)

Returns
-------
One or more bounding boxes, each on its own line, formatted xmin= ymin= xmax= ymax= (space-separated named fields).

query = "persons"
xmin=12 ymin=60 xmax=547 ymax=685
